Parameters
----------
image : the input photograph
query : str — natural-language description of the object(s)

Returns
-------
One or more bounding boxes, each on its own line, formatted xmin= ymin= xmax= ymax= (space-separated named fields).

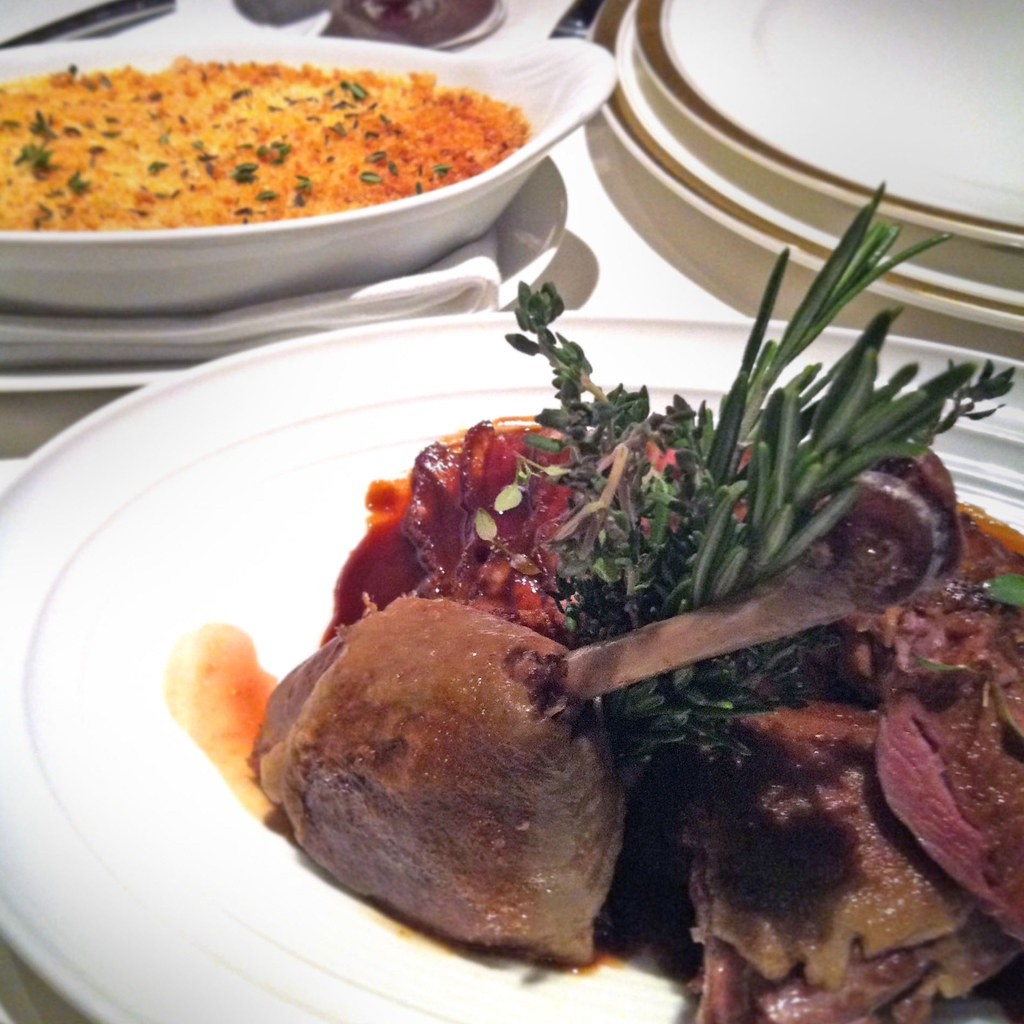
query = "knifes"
xmin=550 ymin=0 xmax=605 ymax=41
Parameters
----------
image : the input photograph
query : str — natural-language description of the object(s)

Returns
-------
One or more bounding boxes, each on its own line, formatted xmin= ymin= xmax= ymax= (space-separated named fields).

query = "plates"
xmin=589 ymin=0 xmax=1024 ymax=335
xmin=0 ymin=312 xmax=1024 ymax=1024
xmin=635 ymin=0 xmax=1024 ymax=253
xmin=0 ymin=150 xmax=567 ymax=391
xmin=0 ymin=36 xmax=617 ymax=319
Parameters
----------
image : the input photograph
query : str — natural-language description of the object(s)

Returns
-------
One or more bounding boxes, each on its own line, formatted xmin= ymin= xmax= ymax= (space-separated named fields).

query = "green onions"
xmin=0 ymin=65 xmax=448 ymax=203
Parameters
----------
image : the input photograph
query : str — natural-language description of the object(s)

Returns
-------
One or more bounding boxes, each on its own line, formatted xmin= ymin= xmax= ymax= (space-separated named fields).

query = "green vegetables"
xmin=503 ymin=181 xmax=1014 ymax=778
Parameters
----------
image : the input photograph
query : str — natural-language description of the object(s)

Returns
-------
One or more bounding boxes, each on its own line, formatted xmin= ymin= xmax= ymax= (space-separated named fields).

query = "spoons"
xmin=331 ymin=0 xmax=507 ymax=51
